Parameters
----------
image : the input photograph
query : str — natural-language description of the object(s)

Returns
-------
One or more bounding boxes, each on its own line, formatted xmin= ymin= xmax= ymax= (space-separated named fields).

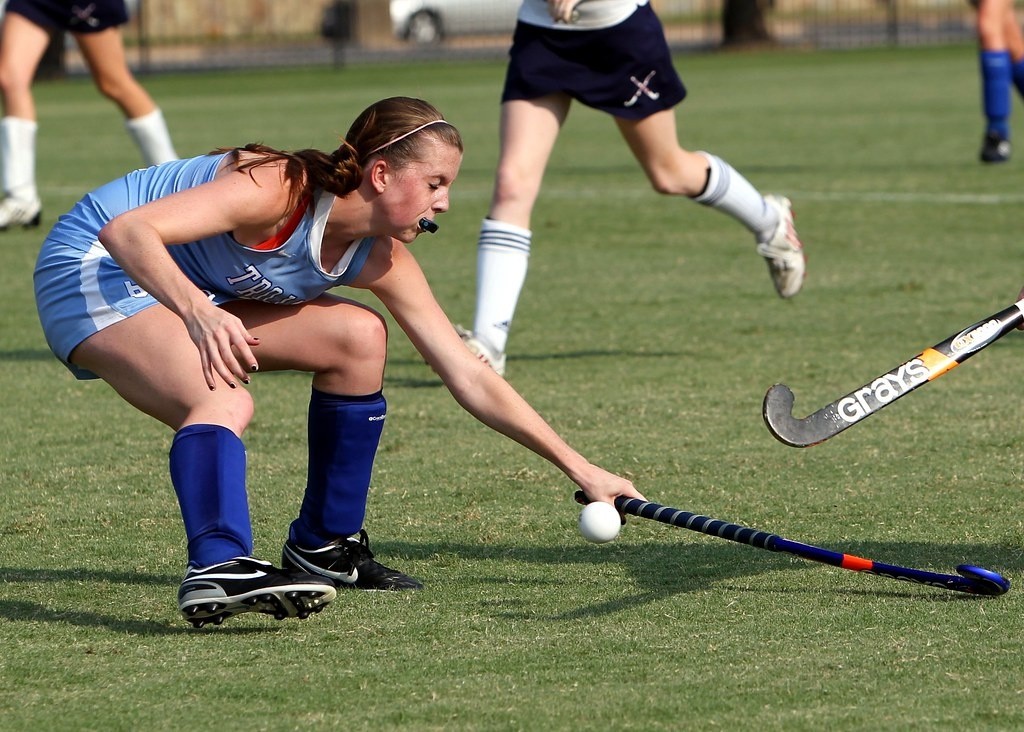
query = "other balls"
xmin=577 ymin=501 xmax=622 ymax=543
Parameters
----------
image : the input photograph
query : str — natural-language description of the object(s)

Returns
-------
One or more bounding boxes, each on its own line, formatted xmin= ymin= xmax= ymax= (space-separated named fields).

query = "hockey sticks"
xmin=573 ymin=488 xmax=1012 ymax=598
xmin=763 ymin=299 xmax=1024 ymax=449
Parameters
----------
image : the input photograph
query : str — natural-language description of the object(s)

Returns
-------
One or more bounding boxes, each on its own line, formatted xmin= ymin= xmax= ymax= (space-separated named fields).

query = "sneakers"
xmin=281 ymin=518 xmax=425 ymax=594
xmin=178 ymin=556 xmax=336 ymax=628
xmin=424 ymin=324 xmax=506 ymax=376
xmin=756 ymin=195 xmax=808 ymax=298
xmin=0 ymin=197 xmax=41 ymax=231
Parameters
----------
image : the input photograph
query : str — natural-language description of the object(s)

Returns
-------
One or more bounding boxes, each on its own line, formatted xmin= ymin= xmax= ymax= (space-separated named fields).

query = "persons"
xmin=958 ymin=0 xmax=1024 ymax=164
xmin=422 ymin=0 xmax=807 ymax=382
xmin=0 ymin=0 xmax=181 ymax=234
xmin=314 ymin=0 xmax=360 ymax=75
xmin=32 ymin=97 xmax=649 ymax=632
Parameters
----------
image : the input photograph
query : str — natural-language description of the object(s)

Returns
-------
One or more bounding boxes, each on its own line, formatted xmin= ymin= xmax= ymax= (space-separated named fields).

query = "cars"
xmin=390 ymin=0 xmax=525 ymax=50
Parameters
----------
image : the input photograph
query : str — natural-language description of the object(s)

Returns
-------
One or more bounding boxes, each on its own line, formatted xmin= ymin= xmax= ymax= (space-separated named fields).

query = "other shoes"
xmin=979 ymin=130 xmax=1010 ymax=163
